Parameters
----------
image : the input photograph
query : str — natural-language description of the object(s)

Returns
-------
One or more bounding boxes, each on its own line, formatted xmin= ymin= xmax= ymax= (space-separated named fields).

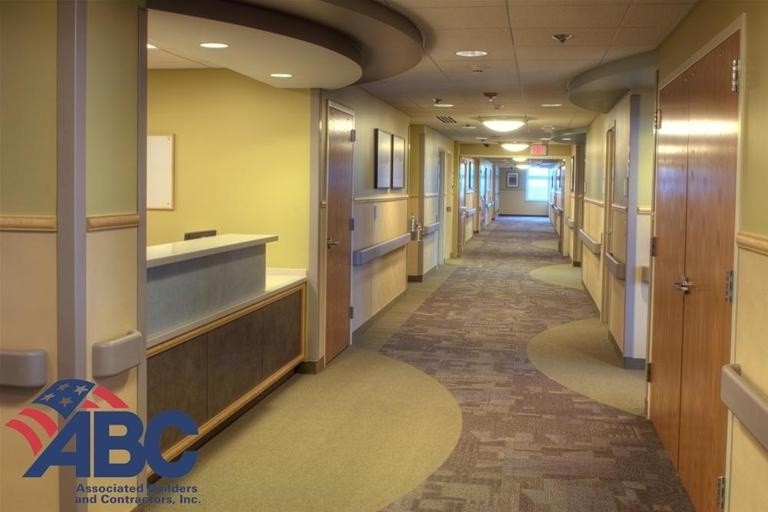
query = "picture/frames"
xmin=506 ymin=172 xmax=519 ymax=188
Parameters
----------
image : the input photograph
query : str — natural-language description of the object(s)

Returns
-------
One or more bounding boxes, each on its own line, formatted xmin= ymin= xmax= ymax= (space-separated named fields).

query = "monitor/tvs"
xmin=185 ymin=229 xmax=217 ymax=242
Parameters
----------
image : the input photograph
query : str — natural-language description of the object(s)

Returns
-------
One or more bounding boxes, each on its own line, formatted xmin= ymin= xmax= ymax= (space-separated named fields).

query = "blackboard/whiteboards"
xmin=145 ymin=135 xmax=176 ymax=211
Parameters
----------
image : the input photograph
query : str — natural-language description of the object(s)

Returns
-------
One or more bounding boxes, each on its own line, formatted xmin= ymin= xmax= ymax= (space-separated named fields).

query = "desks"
xmin=147 ymin=282 xmax=308 ymax=485
